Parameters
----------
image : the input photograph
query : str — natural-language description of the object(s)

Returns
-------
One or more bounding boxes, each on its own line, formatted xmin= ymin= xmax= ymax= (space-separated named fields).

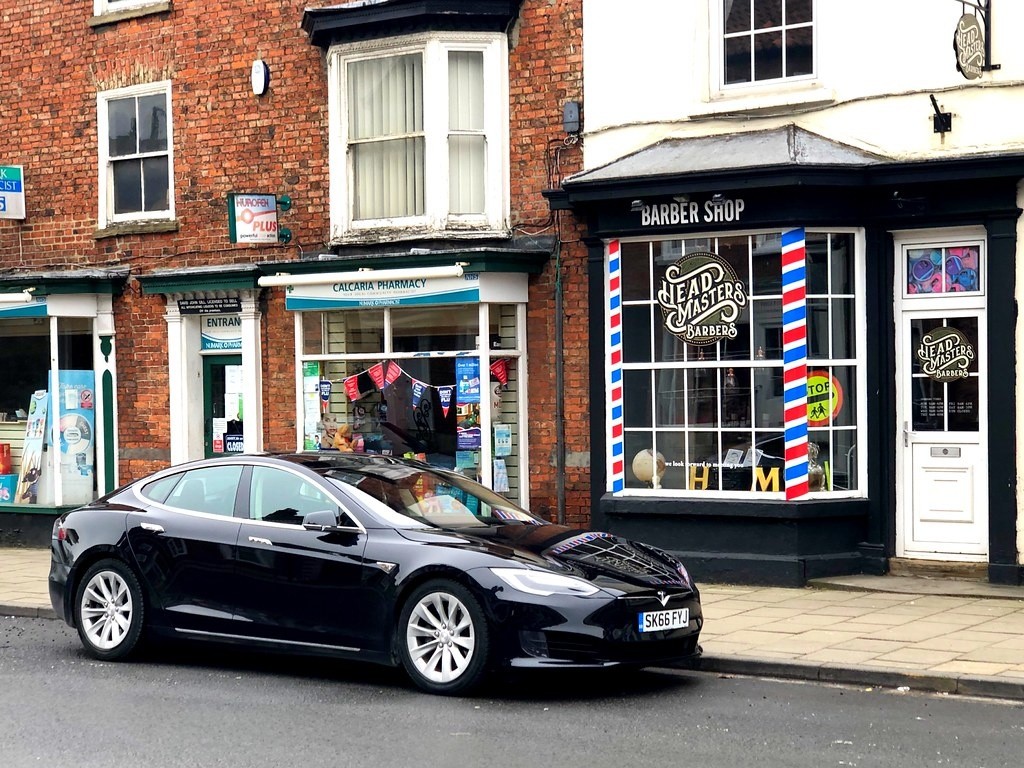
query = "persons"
xmin=320 ymin=414 xmax=338 ymax=448
xmin=313 ymin=434 xmax=321 ymax=449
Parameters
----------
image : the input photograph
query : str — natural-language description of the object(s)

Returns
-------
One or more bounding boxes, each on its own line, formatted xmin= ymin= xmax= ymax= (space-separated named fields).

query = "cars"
xmin=323 ymin=416 xmax=457 ymax=473
xmin=696 ymin=429 xmax=786 ymax=494
xmin=48 ymin=448 xmax=706 ymax=699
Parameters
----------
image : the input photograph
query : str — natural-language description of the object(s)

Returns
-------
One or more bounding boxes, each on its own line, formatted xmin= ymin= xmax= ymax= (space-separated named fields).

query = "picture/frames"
xmin=723 ymin=448 xmax=744 ymax=464
xmin=743 ymin=448 xmax=763 ymax=465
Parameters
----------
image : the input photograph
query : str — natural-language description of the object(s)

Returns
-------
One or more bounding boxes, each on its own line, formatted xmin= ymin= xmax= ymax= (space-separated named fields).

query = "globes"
xmin=633 ymin=448 xmax=665 ymax=489
